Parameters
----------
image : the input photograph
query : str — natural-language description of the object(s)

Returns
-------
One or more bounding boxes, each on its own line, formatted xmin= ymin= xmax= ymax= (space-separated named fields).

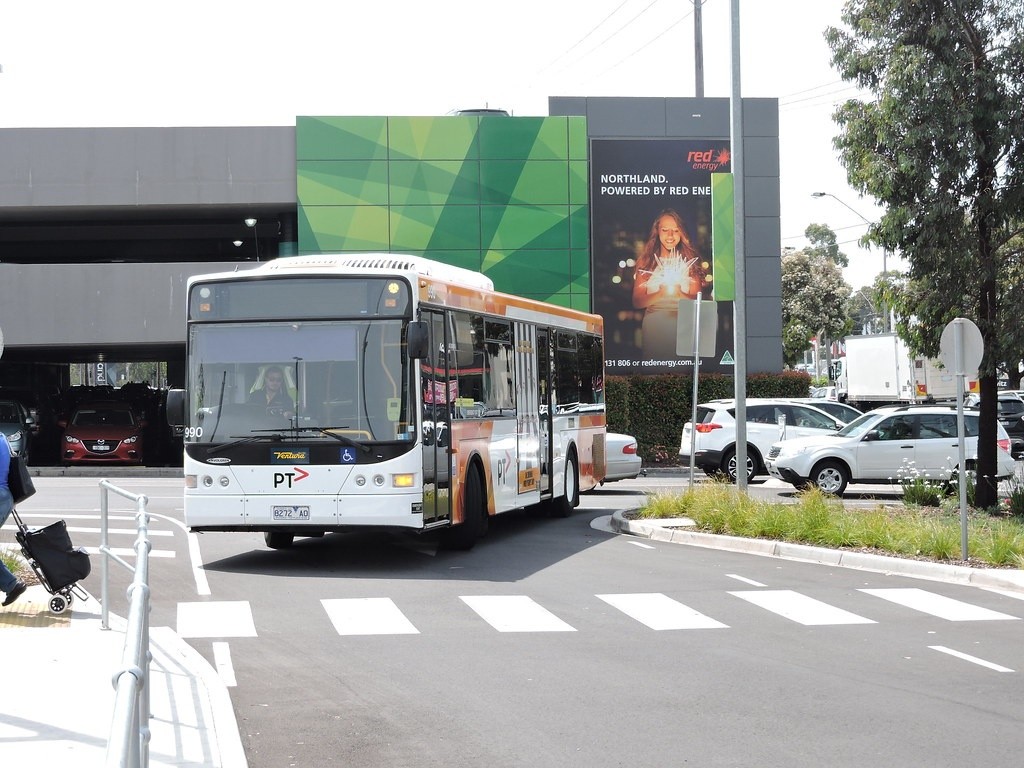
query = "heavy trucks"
xmin=827 ymin=332 xmax=970 ymax=411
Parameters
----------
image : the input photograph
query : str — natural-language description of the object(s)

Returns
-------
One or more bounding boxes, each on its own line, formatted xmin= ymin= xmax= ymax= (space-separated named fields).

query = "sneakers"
xmin=2 ymin=582 xmax=27 ymax=606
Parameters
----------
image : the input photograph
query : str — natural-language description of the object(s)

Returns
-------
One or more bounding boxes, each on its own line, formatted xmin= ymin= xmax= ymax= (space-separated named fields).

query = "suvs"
xmin=763 ymin=404 xmax=1017 ymax=506
xmin=710 ymin=399 xmax=866 ymax=425
xmin=963 ymin=389 xmax=1024 ymax=460
xmin=678 ymin=401 xmax=851 ymax=484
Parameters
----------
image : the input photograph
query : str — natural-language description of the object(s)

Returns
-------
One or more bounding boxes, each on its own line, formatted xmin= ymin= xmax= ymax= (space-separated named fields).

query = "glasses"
xmin=267 ymin=376 xmax=282 ymax=382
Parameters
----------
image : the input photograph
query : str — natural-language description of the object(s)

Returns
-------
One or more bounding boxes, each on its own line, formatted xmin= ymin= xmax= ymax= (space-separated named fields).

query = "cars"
xmin=0 ymin=399 xmax=34 ymax=465
xmin=57 ymin=400 xmax=147 ymax=465
xmin=20 ymin=380 xmax=167 ymax=446
xmin=811 ymin=385 xmax=836 ymax=401
xmin=580 ymin=433 xmax=641 ymax=498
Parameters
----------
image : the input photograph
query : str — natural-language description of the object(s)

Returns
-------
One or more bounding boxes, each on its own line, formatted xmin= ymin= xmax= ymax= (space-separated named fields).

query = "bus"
xmin=165 ymin=253 xmax=606 ymax=551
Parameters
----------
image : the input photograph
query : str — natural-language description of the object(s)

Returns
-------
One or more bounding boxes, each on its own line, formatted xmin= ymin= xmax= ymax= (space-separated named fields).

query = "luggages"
xmin=11 ymin=508 xmax=91 ymax=614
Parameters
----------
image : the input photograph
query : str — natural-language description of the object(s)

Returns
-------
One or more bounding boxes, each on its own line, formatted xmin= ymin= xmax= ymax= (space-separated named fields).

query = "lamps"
xmin=245 ymin=218 xmax=257 ymax=227
xmin=233 ymin=240 xmax=243 ymax=246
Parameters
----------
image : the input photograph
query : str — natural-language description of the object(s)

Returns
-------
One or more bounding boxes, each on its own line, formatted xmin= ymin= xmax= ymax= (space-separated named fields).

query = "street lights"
xmin=812 ymin=191 xmax=889 ymax=332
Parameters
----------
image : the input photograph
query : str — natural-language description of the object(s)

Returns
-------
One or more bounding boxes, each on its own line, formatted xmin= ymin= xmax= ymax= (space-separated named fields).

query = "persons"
xmin=0 ymin=429 xmax=27 ymax=606
xmin=247 ymin=366 xmax=296 ymax=417
xmin=632 ymin=205 xmax=702 ymax=376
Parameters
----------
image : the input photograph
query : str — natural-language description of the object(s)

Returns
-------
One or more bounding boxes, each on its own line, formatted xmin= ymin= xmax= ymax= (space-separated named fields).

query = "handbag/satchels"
xmin=2 ymin=433 xmax=36 ymax=505
